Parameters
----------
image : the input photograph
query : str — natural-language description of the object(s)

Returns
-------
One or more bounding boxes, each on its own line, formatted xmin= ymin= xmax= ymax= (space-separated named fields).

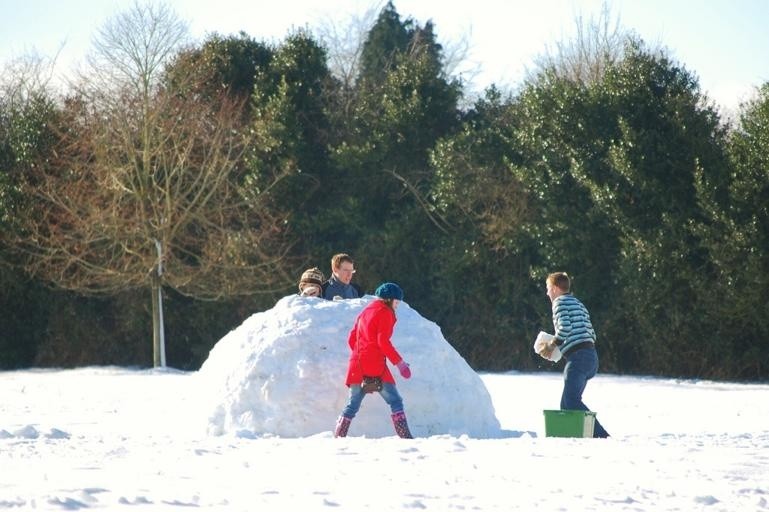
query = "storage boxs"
xmin=544 ymin=409 xmax=596 ymax=437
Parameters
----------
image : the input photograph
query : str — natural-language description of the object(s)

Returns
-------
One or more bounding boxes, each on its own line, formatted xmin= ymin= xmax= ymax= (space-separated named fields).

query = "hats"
xmin=300 ymin=266 xmax=323 ymax=292
xmin=375 ymin=283 xmax=403 ymax=300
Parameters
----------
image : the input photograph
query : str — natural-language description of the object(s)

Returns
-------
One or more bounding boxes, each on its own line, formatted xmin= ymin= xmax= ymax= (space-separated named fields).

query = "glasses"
xmin=342 ymin=269 xmax=355 ymax=274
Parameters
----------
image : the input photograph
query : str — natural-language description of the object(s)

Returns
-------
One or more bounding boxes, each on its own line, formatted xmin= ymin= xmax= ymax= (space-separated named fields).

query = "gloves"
xmin=303 ymin=287 xmax=316 ymax=295
xmin=396 ymin=359 xmax=410 ymax=378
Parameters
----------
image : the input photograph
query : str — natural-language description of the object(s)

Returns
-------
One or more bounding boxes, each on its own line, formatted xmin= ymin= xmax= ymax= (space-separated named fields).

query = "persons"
xmin=323 ymin=253 xmax=363 ymax=301
xmin=537 ymin=272 xmax=611 ymax=439
xmin=335 ymin=282 xmax=416 ymax=441
xmin=301 ymin=265 xmax=326 ymax=299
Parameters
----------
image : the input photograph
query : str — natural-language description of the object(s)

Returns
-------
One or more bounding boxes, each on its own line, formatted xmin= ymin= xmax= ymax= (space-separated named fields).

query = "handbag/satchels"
xmin=360 ymin=377 xmax=382 ymax=393
